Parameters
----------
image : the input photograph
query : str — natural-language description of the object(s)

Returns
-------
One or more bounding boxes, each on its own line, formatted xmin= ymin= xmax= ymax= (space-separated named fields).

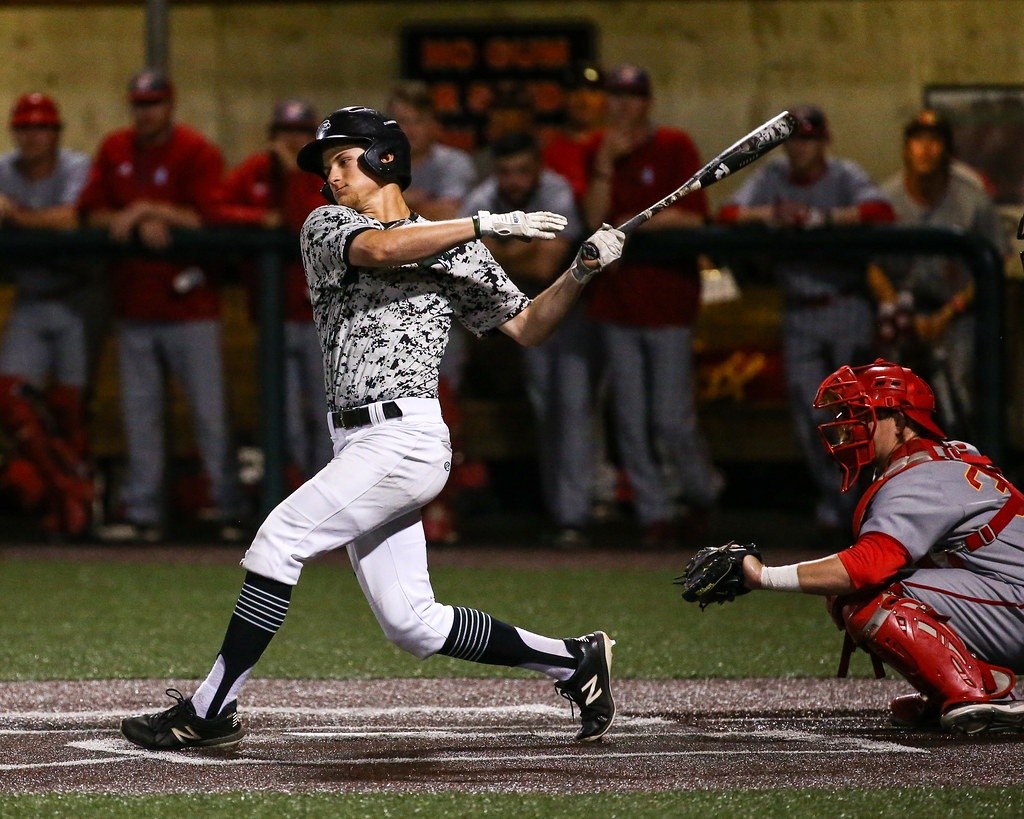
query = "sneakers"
xmin=891 ymin=691 xmax=938 ymax=725
xmin=121 ymin=688 xmax=245 ymax=751
xmin=553 ymin=631 xmax=617 ymax=741
xmin=947 ymin=698 xmax=1024 ymax=745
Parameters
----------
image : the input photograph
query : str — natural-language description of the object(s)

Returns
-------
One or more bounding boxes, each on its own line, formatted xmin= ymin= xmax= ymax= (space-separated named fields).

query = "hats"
xmin=9 ymin=93 xmax=63 ymax=129
xmin=606 ymin=64 xmax=650 ymax=98
xmin=125 ymin=71 xmax=179 ymax=105
xmin=268 ymin=101 xmax=317 ymax=134
xmin=904 ymin=109 xmax=955 ymax=141
xmin=790 ymin=104 xmax=833 ymax=141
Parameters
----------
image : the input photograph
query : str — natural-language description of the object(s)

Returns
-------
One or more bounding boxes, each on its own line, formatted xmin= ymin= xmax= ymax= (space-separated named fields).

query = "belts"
xmin=332 ymin=403 xmax=402 ymax=429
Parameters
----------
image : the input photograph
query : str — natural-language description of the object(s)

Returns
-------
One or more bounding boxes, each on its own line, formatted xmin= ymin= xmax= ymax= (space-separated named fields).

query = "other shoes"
xmin=19 ymin=504 xmax=761 ymax=555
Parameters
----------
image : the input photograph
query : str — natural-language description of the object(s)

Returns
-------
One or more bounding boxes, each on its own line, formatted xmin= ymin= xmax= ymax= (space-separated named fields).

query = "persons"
xmin=684 ymin=361 xmax=1024 ymax=733
xmin=0 ymin=74 xmax=335 ymax=540
xmin=121 ymin=105 xmax=615 ymax=752
xmin=394 ymin=62 xmax=995 ymax=548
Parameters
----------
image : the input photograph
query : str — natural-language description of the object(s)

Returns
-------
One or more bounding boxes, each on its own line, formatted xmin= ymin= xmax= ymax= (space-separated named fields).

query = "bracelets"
xmin=472 ymin=215 xmax=482 ymax=239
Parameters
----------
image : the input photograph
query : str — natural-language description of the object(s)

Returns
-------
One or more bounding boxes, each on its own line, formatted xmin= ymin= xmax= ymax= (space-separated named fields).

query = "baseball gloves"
xmin=680 ymin=542 xmax=767 ymax=607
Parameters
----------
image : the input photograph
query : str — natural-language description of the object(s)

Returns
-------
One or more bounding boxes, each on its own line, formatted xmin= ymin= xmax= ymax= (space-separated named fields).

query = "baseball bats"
xmin=580 ymin=110 xmax=802 ymax=264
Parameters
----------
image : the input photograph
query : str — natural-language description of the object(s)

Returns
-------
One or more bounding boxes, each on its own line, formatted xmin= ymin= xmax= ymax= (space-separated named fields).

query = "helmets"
xmin=298 ymin=105 xmax=411 ymax=204
xmin=813 ymin=359 xmax=945 ymax=492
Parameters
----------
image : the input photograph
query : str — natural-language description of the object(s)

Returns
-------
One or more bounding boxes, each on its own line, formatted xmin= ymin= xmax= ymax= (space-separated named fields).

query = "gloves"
xmin=587 ymin=222 xmax=625 ymax=268
xmin=479 ymin=211 xmax=568 ymax=239
